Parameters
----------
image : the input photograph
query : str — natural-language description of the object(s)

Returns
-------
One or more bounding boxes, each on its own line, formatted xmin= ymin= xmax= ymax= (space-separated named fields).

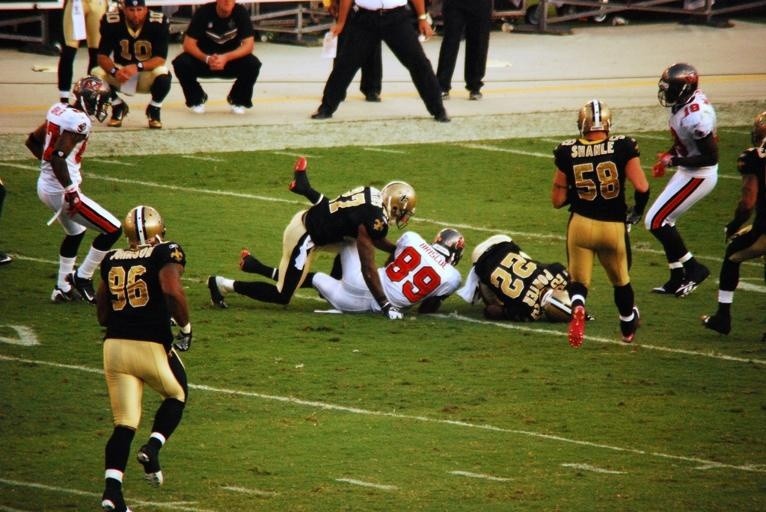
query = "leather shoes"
xmin=311 ymin=110 xmax=333 ymax=120
xmin=432 ymin=111 xmax=451 ymax=125
xmin=365 ymin=91 xmax=382 ymax=102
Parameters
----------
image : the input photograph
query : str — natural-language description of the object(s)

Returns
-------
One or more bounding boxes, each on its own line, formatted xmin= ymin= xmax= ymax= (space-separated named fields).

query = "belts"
xmin=353 ymin=4 xmax=406 ymax=14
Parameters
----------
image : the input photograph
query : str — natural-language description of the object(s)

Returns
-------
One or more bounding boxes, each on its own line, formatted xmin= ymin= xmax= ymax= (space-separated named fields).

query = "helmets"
xmin=68 ymin=77 xmax=111 ymax=122
xmin=433 ymin=226 xmax=466 ymax=267
xmin=657 ymin=64 xmax=699 ymax=114
xmin=121 ymin=203 xmax=169 ymax=250
xmin=542 ymin=289 xmax=572 ymax=320
xmin=379 ymin=179 xmax=419 ymax=232
xmin=751 ymin=113 xmax=766 ymax=149
xmin=576 ymin=97 xmax=614 ymax=137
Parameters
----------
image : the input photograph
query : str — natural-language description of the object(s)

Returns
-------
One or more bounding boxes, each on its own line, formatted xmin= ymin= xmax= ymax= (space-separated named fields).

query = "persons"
xmin=312 ymin=0 xmax=453 ymax=122
xmin=554 ymin=100 xmax=650 ymax=349
xmin=206 ymin=180 xmax=417 ymax=318
xmin=91 ymin=0 xmax=173 ymax=128
xmin=330 ymin=1 xmax=383 ymax=101
xmin=436 ymin=0 xmax=493 ymax=99
xmin=455 ymin=234 xmax=570 ymax=326
xmin=646 ymin=63 xmax=719 ymax=299
xmin=1 ymin=179 xmax=12 ymax=264
xmin=96 ymin=205 xmax=192 ymax=512
xmin=57 ymin=0 xmax=112 ymax=104
xmin=172 ymin=0 xmax=262 ymax=115
xmin=240 ymin=156 xmax=464 ymax=313
xmin=700 ymin=112 xmax=766 ymax=335
xmin=26 ymin=77 xmax=122 ymax=306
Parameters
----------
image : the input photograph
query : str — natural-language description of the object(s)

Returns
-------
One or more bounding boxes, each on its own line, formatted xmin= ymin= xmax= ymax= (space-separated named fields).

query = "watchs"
xmin=205 ymin=54 xmax=211 ymax=64
xmin=419 ymin=14 xmax=427 ymax=20
xmin=109 ymin=68 xmax=118 ymax=77
xmin=137 ymin=61 xmax=144 ymax=72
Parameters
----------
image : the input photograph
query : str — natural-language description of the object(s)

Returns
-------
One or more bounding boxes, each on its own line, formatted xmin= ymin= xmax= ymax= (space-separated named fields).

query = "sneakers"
xmin=470 ymin=90 xmax=481 ymax=102
xmin=188 ymin=94 xmax=206 ymax=114
xmin=105 ymin=97 xmax=129 ymax=126
xmin=136 ymin=444 xmax=164 ymax=490
xmin=567 ymin=305 xmax=585 ymax=349
xmin=51 ymin=284 xmax=88 ymax=305
xmin=99 ymin=489 xmax=133 ymax=512
xmin=652 ymin=276 xmax=678 ymax=298
xmin=618 ymin=304 xmax=641 ymax=342
xmin=673 ymin=261 xmax=710 ymax=299
xmin=0 ymin=253 xmax=13 ymax=264
xmin=65 ymin=276 xmax=97 ymax=303
xmin=143 ymin=103 xmax=163 ymax=130
xmin=288 ymin=157 xmax=309 ymax=196
xmin=439 ymin=89 xmax=449 ymax=99
xmin=223 ymin=101 xmax=246 ymax=114
xmin=239 ymin=247 xmax=258 ymax=272
xmin=208 ymin=276 xmax=228 ymax=310
xmin=700 ymin=312 xmax=732 ymax=336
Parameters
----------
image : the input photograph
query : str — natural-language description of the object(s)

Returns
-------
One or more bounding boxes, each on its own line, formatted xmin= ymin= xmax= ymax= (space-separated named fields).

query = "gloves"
xmin=624 ymin=204 xmax=643 ymax=228
xmin=651 ymin=161 xmax=665 ymax=178
xmin=656 ymin=153 xmax=674 ymax=167
xmin=724 ymin=200 xmax=754 ymax=243
xmin=63 ymin=191 xmax=82 ymax=219
xmin=381 ymin=302 xmax=405 ymax=320
xmin=171 ymin=328 xmax=192 ymax=353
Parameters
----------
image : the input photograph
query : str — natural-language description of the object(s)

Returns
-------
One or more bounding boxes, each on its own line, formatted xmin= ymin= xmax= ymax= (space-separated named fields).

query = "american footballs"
xmin=485 ymin=303 xmax=506 ymax=320
